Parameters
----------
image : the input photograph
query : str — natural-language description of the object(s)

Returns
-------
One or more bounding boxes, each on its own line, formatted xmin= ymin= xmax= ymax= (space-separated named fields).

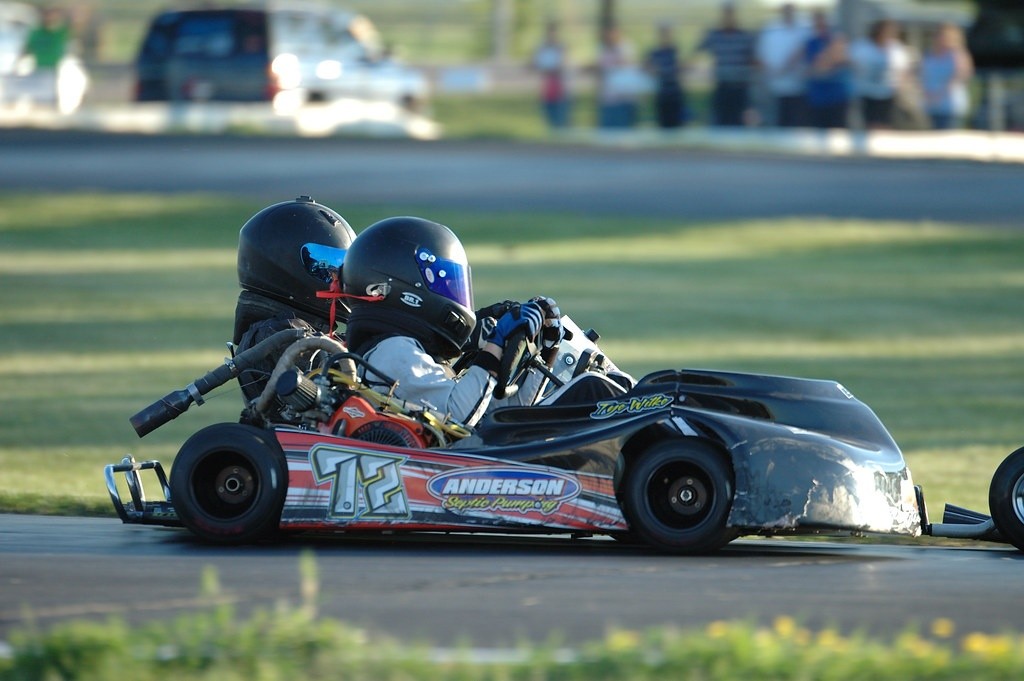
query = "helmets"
xmin=344 ymin=216 xmax=475 ymax=358
xmin=237 ymin=196 xmax=357 ymax=324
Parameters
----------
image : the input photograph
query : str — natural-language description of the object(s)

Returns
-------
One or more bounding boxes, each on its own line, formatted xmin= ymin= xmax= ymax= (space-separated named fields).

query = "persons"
xmin=344 ymin=216 xmax=544 ymax=450
xmin=233 ymin=195 xmax=356 ymax=406
xmin=14 ymin=4 xmax=86 ymax=114
xmin=527 ymin=2 xmax=978 ymax=132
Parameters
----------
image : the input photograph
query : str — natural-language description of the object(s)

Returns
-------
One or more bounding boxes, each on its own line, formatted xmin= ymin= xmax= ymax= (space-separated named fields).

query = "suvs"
xmin=127 ymin=7 xmax=423 ymax=112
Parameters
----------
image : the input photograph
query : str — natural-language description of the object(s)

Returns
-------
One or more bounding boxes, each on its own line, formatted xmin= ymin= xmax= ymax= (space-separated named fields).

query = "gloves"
xmin=487 ymin=304 xmax=546 ymax=353
xmin=528 ymin=295 xmax=564 ymax=349
xmin=474 ymin=303 xmax=507 ymax=321
xmin=461 ymin=316 xmax=497 ymax=352
